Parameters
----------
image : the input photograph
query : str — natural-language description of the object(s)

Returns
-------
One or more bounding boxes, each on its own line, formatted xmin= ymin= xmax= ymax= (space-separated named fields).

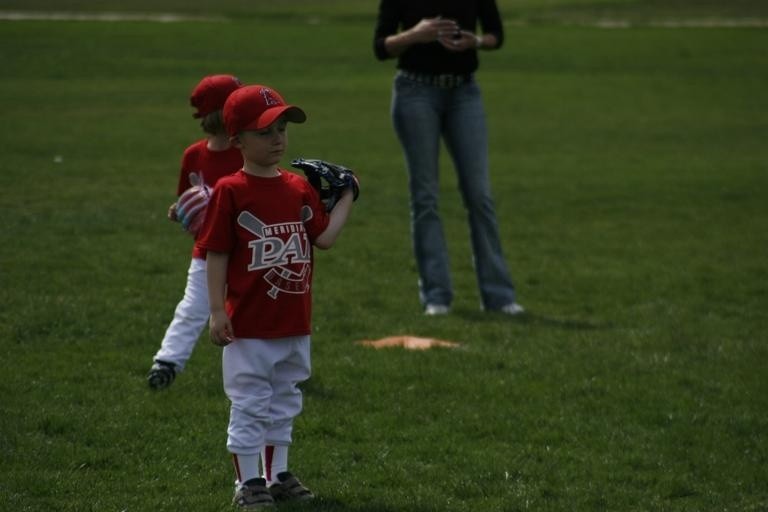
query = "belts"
xmin=398 ymin=69 xmax=472 ymax=89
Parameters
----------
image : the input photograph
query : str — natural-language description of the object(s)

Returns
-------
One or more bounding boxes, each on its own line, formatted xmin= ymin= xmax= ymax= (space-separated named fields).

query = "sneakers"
xmin=233 ymin=478 xmax=275 ymax=508
xmin=147 ymin=366 xmax=177 ymax=390
xmin=268 ymin=471 xmax=315 ymax=500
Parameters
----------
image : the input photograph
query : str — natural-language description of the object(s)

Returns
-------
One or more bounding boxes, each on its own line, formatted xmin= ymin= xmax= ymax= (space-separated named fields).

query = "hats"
xmin=222 ymin=85 xmax=307 ymax=137
xmin=189 ymin=75 xmax=246 ymax=118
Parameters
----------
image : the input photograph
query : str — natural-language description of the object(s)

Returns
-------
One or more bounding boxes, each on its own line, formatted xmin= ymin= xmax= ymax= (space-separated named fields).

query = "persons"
xmin=200 ymin=85 xmax=359 ymax=510
xmin=373 ymin=1 xmax=524 ymax=316
xmin=148 ymin=74 xmax=241 ymax=389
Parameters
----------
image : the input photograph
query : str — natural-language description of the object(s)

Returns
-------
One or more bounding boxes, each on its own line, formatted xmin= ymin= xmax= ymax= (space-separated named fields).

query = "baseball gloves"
xmin=174 ymin=186 xmax=214 ymax=240
xmin=290 ymin=158 xmax=358 ymax=213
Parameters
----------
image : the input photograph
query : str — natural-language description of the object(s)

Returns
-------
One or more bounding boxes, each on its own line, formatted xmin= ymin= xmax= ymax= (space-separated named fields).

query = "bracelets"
xmin=476 ymin=36 xmax=482 ymax=48
